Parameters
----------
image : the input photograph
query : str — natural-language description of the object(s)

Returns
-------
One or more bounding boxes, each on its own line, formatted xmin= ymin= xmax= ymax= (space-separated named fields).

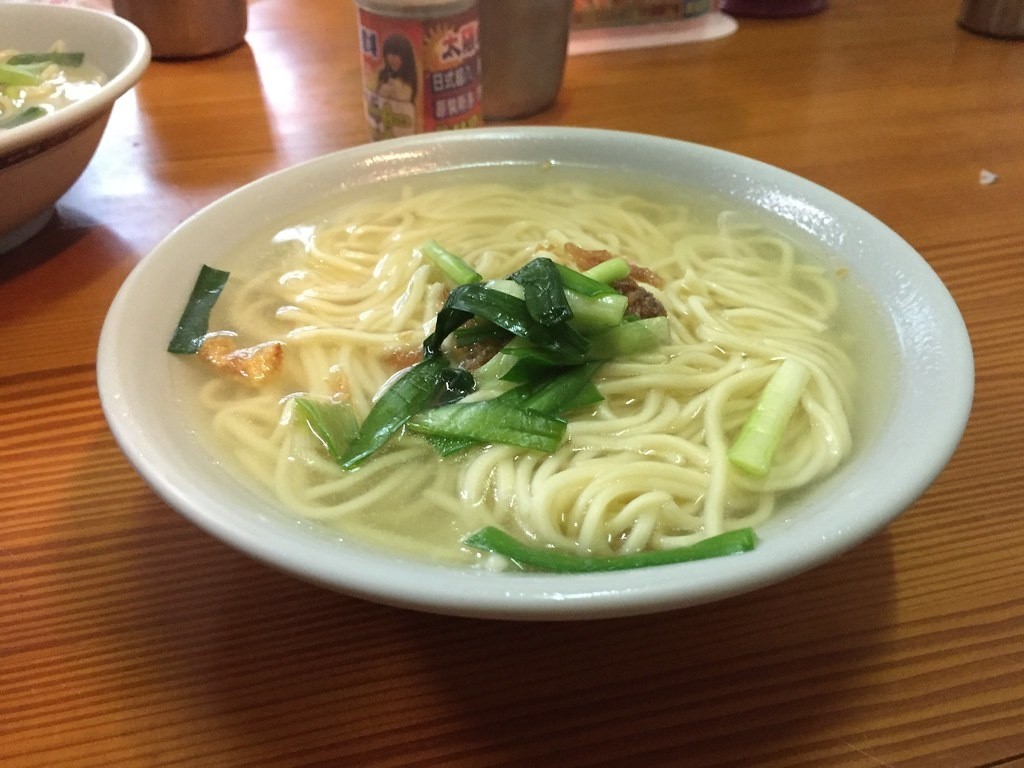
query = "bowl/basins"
xmin=0 ymin=2 xmax=152 ymax=256
xmin=95 ymin=124 xmax=978 ymax=624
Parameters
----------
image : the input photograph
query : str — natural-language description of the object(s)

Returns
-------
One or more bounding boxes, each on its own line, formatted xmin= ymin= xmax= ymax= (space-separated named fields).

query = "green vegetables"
xmin=0 ymin=49 xmax=88 ymax=130
xmin=170 ymin=244 xmax=767 ymax=574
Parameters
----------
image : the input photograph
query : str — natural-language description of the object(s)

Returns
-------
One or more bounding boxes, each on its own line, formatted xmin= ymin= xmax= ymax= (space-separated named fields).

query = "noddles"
xmin=0 ymin=38 xmax=110 ymax=131
xmin=170 ymin=171 xmax=900 ymax=572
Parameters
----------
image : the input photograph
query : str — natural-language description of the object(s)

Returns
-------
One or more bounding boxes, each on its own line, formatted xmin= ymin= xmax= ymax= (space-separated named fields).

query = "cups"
xmin=479 ymin=1 xmax=571 ymax=122
xmin=111 ymin=0 xmax=247 ymax=60
xmin=956 ymin=0 xmax=1024 ymax=39
